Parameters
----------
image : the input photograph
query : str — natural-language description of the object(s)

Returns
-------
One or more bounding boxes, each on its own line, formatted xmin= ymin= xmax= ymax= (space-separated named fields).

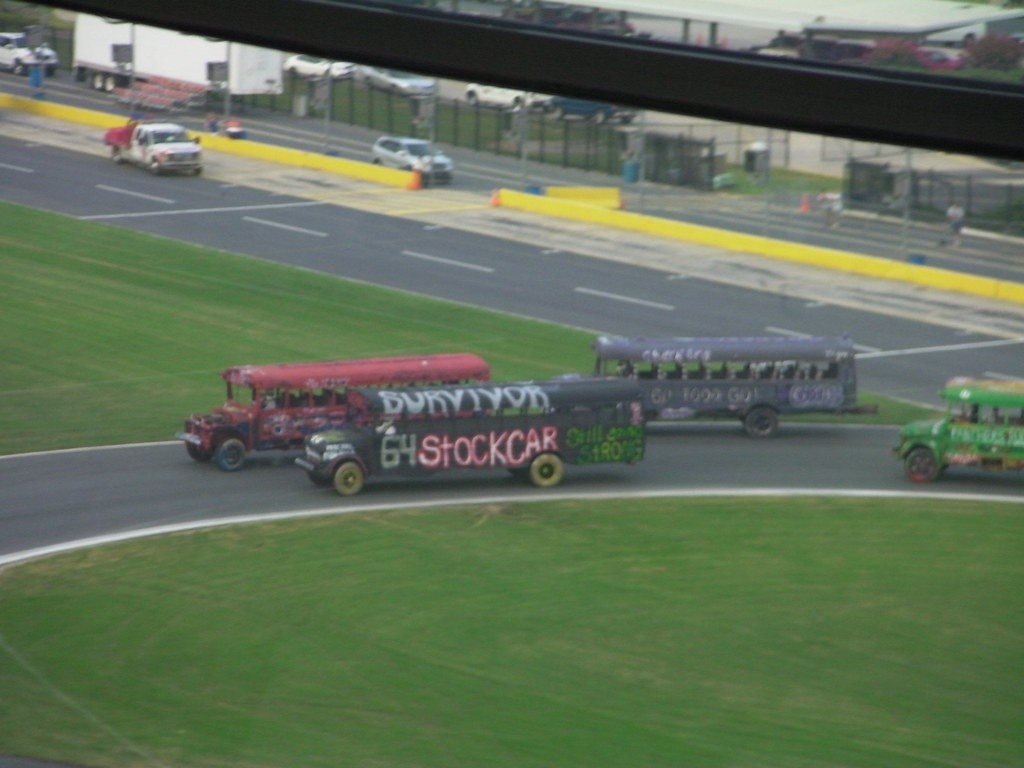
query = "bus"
xmin=173 ymin=352 xmax=490 ymax=471
xmin=293 ymin=377 xmax=649 ymax=497
xmin=544 ymin=332 xmax=879 ymax=438
xmin=891 ymin=376 xmax=1023 ymax=487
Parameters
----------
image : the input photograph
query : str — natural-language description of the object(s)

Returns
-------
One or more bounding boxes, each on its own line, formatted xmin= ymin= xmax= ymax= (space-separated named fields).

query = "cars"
xmin=359 ymin=64 xmax=437 ymax=95
xmin=283 ymin=52 xmax=355 ymax=82
xmin=543 ymin=96 xmax=637 ymax=125
xmin=465 ymin=83 xmax=550 ymax=114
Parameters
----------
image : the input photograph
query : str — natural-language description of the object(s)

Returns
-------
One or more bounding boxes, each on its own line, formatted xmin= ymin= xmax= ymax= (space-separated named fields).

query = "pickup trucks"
xmin=111 ymin=119 xmax=204 ymax=178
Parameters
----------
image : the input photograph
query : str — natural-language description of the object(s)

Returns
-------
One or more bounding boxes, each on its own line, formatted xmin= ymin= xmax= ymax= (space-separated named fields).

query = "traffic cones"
xmin=800 ymin=192 xmax=812 ymax=211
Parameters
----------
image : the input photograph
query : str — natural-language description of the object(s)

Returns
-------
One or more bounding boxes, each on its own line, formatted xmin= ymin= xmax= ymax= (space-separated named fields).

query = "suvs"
xmin=369 ymin=134 xmax=454 ymax=184
xmin=0 ymin=32 xmax=59 ymax=77
